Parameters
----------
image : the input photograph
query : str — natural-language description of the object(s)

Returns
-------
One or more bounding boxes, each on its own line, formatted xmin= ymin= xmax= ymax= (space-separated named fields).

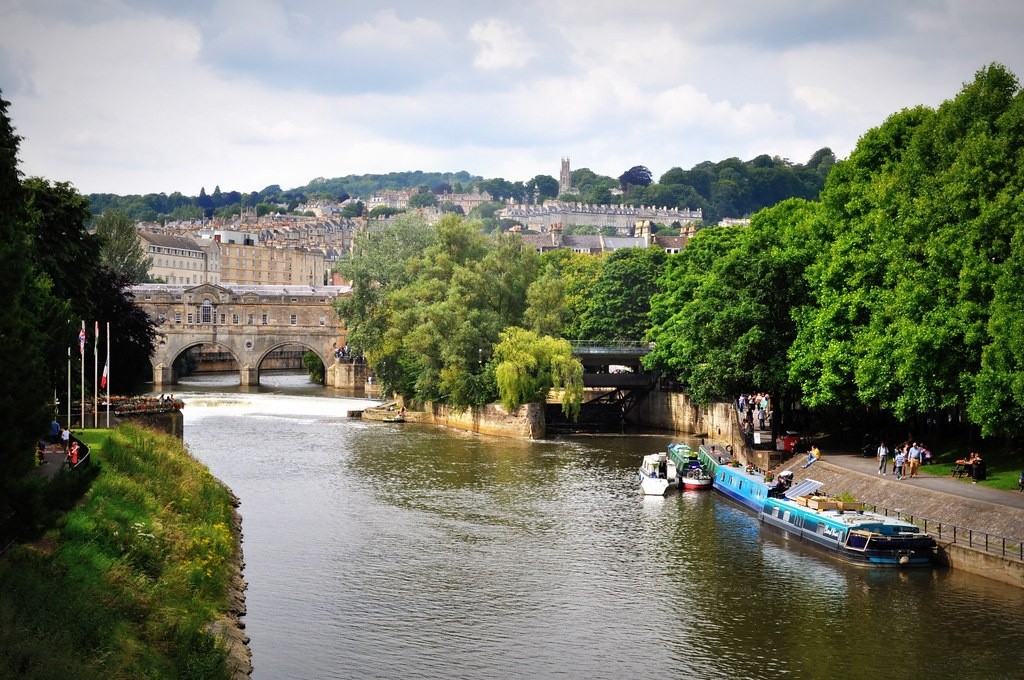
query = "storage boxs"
xmin=831 ymin=497 xmax=864 ymax=510
xmin=808 ymin=499 xmax=837 ymax=510
xmin=797 ymin=494 xmax=826 ymax=507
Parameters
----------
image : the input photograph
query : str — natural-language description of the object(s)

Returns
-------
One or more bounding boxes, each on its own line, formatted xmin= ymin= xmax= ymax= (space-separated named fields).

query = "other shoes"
xmin=52 ymin=452 xmax=54 ymax=453
xmin=902 ymin=476 xmax=907 ymax=480
xmin=878 ymin=470 xmax=881 ymax=475
xmin=892 ymin=472 xmax=894 ymax=474
xmin=55 ymin=451 xmax=57 ymax=452
xmin=896 ymin=477 xmax=902 ymax=480
xmin=64 ymin=453 xmax=66 ymax=454
xmin=972 ymin=482 xmax=977 ymax=484
xmin=965 ymin=473 xmax=968 ymax=476
xmin=801 ymin=466 xmax=806 ymax=468
xmin=910 ymin=476 xmax=912 ymax=478
xmin=883 ymin=473 xmax=887 ymax=476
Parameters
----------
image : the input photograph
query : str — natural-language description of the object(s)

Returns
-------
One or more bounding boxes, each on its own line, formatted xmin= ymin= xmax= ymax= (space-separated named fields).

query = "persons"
xmin=596 ymin=364 xmax=607 ymax=374
xmin=876 ymin=441 xmax=934 ymax=480
xmin=398 ymin=407 xmax=406 ymax=418
xmin=60 ymin=426 xmax=70 ymax=454
xmin=801 ymin=444 xmax=821 ymax=468
xmin=38 ymin=439 xmax=48 ymax=465
xmin=738 ymin=392 xmax=769 ymax=430
xmin=67 ymin=441 xmax=80 ymax=470
xmin=158 ymin=394 xmax=174 ymax=403
xmin=334 ymin=344 xmax=364 ymax=364
xmin=965 ymin=452 xmax=983 ymax=485
xmin=49 ymin=416 xmax=60 ymax=453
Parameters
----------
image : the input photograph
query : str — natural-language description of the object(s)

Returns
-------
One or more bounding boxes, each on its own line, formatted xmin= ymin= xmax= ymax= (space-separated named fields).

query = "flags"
xmin=93 ymin=326 xmax=100 ymax=355
xmin=100 ymin=359 xmax=108 ymax=389
xmin=79 ymin=329 xmax=86 ymax=352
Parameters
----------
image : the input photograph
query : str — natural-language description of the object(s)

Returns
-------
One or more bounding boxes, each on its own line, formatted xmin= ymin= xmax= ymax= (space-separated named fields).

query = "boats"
xmin=638 ymin=452 xmax=676 ymax=496
xmin=698 ymin=444 xmax=743 ymax=481
xmin=757 ymin=478 xmax=940 ymax=570
xmin=713 ymin=462 xmax=790 ymax=512
xmin=667 ymin=443 xmax=712 ymax=492
xmin=361 ymin=407 xmax=406 ymax=423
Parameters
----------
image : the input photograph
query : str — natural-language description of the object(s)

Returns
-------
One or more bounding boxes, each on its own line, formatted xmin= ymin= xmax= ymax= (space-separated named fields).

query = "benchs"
xmin=951 ymin=458 xmax=970 ymax=478
